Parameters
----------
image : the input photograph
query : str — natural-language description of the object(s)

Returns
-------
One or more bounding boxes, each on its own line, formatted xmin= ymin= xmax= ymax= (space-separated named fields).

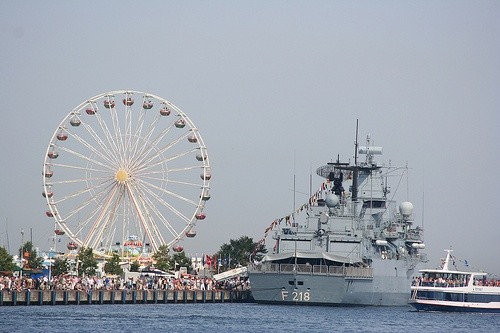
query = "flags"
xmin=188 ymin=253 xmax=223 ymax=269
xmin=249 ymin=179 xmax=332 ymax=256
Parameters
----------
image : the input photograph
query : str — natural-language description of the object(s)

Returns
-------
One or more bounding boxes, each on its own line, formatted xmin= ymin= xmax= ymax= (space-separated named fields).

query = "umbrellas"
xmin=453 ymin=259 xmax=468 ymax=266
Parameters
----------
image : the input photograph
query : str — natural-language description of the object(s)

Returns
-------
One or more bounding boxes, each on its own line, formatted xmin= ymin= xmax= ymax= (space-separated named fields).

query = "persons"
xmin=412 ymin=275 xmax=500 ymax=289
xmin=0 ymin=275 xmax=249 ymax=295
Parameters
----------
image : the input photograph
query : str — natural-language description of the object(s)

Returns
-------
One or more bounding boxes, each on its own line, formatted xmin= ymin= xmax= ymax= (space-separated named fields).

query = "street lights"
xmin=20 ymin=230 xmax=24 ymax=279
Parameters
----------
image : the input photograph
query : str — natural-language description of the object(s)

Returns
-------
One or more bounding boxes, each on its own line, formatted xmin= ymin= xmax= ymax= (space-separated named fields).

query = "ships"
xmin=246 ymin=117 xmax=429 ymax=308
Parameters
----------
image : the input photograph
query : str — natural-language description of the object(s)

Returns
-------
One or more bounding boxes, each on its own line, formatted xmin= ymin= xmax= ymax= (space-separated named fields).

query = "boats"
xmin=407 ymin=248 xmax=500 ymax=313
